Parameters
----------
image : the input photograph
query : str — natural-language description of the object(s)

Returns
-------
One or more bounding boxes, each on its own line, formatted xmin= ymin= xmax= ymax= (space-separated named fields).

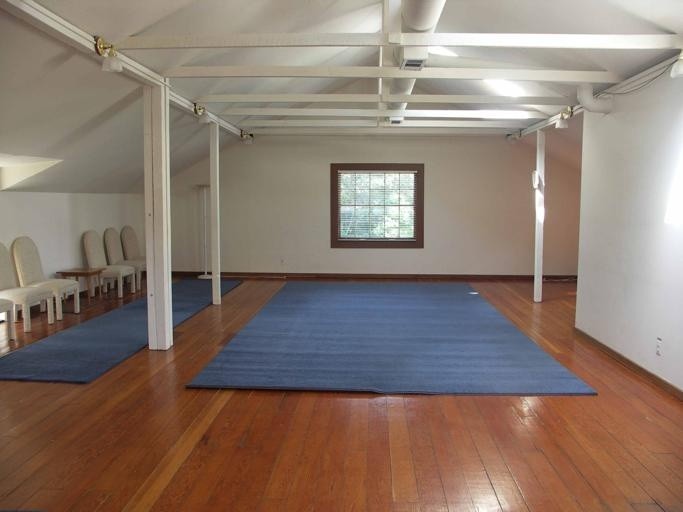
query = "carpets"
xmin=4 ymin=278 xmax=244 ymax=382
xmin=189 ymin=278 xmax=597 ymax=396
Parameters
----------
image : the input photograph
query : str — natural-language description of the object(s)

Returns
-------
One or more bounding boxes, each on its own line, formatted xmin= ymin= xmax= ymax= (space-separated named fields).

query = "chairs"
xmin=0 ymin=225 xmax=146 ymax=342
